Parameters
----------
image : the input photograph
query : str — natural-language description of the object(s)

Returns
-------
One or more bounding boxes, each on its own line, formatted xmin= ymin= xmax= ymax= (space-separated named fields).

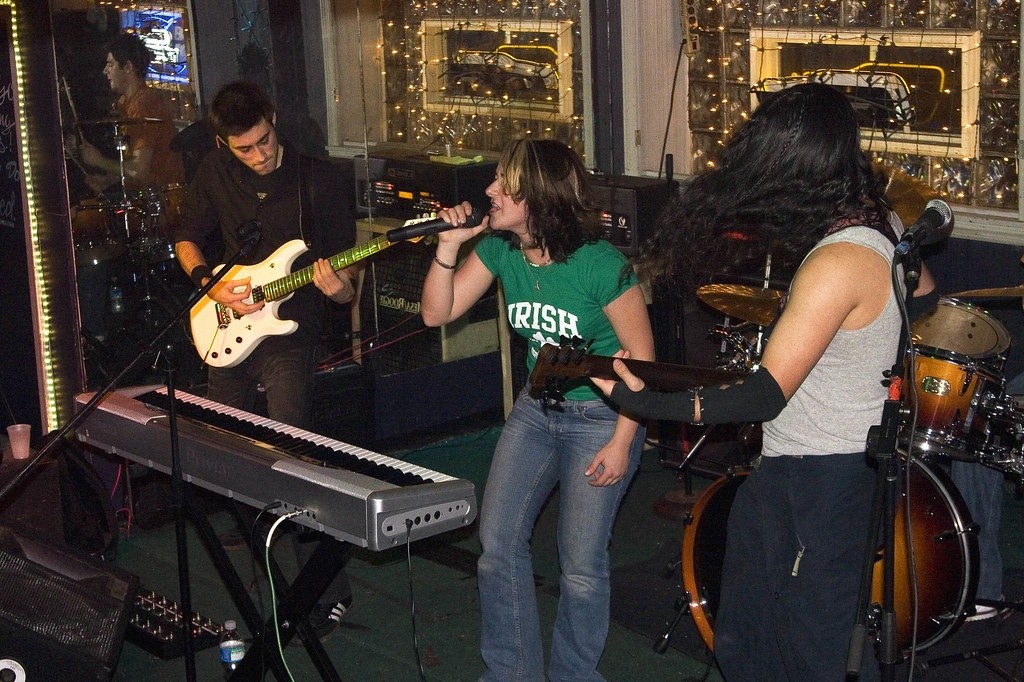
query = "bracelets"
xmin=191 ymin=265 xmax=213 ymax=288
xmin=433 ymin=251 xmax=456 ymax=269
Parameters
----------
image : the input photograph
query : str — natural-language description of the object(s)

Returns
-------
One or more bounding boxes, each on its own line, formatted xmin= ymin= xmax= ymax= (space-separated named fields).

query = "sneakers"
xmin=295 ymin=595 xmax=353 ymax=643
xmin=219 ymin=523 xmax=281 ymax=550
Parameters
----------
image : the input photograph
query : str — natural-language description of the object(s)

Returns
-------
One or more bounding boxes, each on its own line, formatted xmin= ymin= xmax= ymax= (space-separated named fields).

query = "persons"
xmin=173 ymin=84 xmax=359 ymax=645
xmin=80 ymin=33 xmax=184 ymax=226
xmin=590 ymin=83 xmax=938 ymax=682
xmin=421 ymin=137 xmax=654 ymax=682
xmin=938 ymin=373 xmax=1024 ymax=622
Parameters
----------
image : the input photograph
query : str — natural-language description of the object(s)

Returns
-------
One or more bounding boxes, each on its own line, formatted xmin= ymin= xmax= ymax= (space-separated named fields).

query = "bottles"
xmin=221 ymin=619 xmax=245 ymax=680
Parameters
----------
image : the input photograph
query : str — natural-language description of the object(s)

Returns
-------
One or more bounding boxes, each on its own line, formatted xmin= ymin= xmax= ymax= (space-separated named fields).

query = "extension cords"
xmin=679 ymin=0 xmax=701 ymax=55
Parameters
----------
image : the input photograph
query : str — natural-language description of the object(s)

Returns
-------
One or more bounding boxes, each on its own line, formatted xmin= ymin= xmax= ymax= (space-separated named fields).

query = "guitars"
xmin=188 ymin=206 xmax=443 ymax=374
xmin=528 ymin=333 xmax=764 ymax=456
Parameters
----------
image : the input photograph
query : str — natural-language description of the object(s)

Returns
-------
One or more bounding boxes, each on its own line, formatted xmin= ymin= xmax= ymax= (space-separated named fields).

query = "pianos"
xmin=74 ymin=385 xmax=481 ymax=682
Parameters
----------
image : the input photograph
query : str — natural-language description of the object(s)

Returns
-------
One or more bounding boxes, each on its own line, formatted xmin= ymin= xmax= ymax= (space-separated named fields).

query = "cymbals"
xmin=955 ymin=284 xmax=1024 ymax=298
xmin=170 ymin=116 xmax=214 ymax=153
xmin=694 ymin=282 xmax=788 ymax=328
xmin=62 ymin=116 xmax=193 ymax=129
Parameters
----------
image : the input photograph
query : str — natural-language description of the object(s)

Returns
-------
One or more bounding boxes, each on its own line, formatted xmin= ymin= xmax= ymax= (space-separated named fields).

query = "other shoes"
xmin=964 ymin=600 xmax=1022 ymax=622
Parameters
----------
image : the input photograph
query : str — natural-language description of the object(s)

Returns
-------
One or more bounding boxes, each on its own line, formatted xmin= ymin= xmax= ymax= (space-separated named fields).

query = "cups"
xmin=8 ymin=424 xmax=31 ymax=459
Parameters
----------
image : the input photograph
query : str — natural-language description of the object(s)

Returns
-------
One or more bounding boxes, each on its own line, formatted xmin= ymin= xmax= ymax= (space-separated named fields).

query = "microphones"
xmin=892 ymin=198 xmax=952 ymax=257
xmin=387 ymin=207 xmax=483 ymax=243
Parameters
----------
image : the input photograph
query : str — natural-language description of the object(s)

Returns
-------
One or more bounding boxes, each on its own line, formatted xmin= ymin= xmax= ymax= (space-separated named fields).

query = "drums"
xmin=116 ymin=181 xmax=202 ymax=270
xmin=682 ymin=448 xmax=979 ymax=662
xmin=56 ymin=191 xmax=131 ymax=273
xmin=893 ymin=294 xmax=1024 ymax=477
